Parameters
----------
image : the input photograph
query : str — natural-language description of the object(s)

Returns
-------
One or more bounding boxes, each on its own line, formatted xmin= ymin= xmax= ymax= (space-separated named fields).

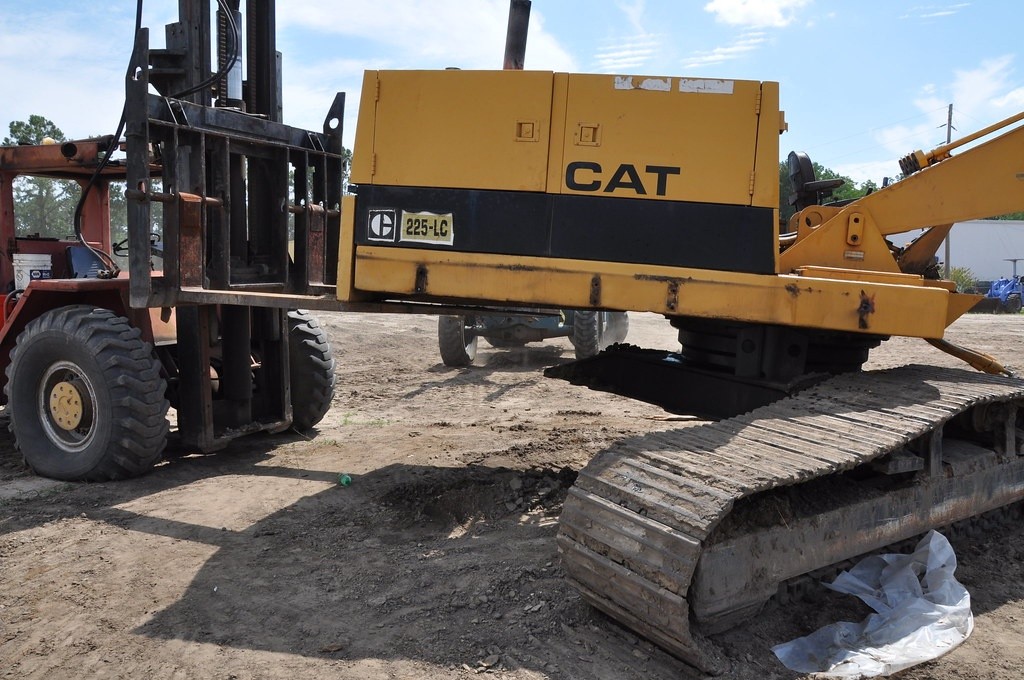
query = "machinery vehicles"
xmin=330 ymin=0 xmax=1024 ymax=679
xmin=0 ymin=1 xmax=347 ymax=486
xmin=438 ymin=298 xmax=630 ymax=366
xmin=967 ymin=275 xmax=1024 ymax=314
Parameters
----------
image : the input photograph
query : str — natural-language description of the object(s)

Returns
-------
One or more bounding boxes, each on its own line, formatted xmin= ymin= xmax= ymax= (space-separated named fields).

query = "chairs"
xmin=65 ymin=246 xmax=107 ymax=279
